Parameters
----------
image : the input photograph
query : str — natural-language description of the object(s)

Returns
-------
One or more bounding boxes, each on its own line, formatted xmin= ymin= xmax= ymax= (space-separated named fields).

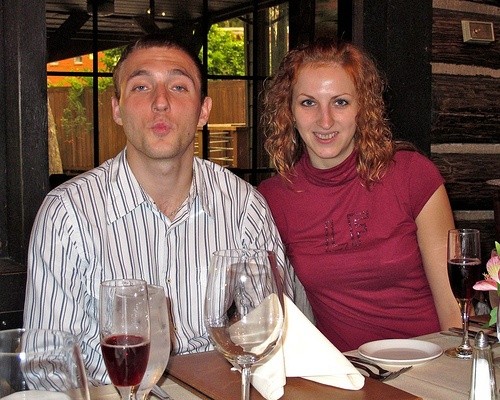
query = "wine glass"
xmin=99 ymin=279 xmax=151 ymax=400
xmin=113 ymin=285 xmax=170 ymax=400
xmin=445 ymin=229 xmax=482 ymax=359
xmin=204 ymin=249 xmax=286 ymax=400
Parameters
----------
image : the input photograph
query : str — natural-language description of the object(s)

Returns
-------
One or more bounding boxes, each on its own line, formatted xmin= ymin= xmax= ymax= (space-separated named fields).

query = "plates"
xmin=358 ymin=339 xmax=444 ymax=367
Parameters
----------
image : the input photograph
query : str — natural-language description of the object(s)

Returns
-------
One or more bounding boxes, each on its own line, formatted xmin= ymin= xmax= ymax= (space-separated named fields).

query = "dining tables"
xmin=79 ymin=324 xmax=500 ymax=400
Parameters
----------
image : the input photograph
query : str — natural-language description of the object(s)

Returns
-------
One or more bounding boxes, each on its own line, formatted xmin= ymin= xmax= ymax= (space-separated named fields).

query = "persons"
xmin=18 ymin=30 xmax=297 ymax=400
xmin=254 ymin=26 xmax=476 ymax=354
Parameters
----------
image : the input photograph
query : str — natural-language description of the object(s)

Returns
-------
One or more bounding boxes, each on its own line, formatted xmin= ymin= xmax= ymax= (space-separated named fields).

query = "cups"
xmin=0 ymin=330 xmax=91 ymax=400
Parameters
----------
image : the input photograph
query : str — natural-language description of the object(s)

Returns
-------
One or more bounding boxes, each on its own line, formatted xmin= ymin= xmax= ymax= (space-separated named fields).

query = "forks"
xmin=350 ymin=362 xmax=400 ymax=382
xmin=345 ymin=355 xmax=412 ymax=380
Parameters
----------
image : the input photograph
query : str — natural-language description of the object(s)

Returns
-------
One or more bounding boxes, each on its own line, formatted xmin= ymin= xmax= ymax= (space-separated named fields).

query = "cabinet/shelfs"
xmin=193 ymin=124 xmax=249 ymax=168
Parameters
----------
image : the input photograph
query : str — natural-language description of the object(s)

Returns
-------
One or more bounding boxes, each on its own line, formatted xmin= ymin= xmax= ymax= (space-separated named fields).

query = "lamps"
xmin=87 ymin=0 xmax=114 ymax=17
